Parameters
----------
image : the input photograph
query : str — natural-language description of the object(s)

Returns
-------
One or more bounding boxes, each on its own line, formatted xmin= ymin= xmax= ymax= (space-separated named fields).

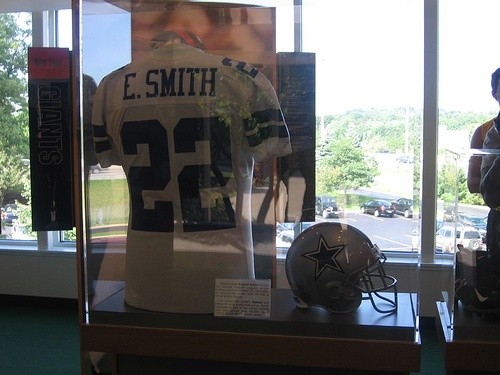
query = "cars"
xmin=359 ymin=199 xmax=397 ymax=218
xmin=0 ymin=203 xmax=19 ymax=226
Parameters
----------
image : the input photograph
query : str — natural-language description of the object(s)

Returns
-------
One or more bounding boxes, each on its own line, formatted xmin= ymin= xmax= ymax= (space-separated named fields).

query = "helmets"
xmin=150 ymin=29 xmax=207 ymax=51
xmin=284 ymin=222 xmax=382 ymax=314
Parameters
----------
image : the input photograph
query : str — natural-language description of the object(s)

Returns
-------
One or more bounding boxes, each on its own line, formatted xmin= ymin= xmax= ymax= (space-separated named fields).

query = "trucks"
xmin=315 ymin=195 xmax=340 ymax=219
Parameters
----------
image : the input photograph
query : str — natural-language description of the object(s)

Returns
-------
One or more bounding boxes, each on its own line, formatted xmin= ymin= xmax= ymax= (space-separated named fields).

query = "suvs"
xmin=391 ymin=198 xmax=415 ymax=217
xmin=434 ymin=210 xmax=489 ymax=253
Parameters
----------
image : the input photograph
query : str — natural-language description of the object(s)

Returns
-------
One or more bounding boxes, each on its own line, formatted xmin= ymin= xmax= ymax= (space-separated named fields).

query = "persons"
xmin=70 ymin=51 xmax=99 ymax=189
xmin=92 ymin=44 xmax=292 ymax=314
xmin=468 ymin=68 xmax=500 ymax=252
xmin=480 ymin=111 xmax=500 ymax=209
